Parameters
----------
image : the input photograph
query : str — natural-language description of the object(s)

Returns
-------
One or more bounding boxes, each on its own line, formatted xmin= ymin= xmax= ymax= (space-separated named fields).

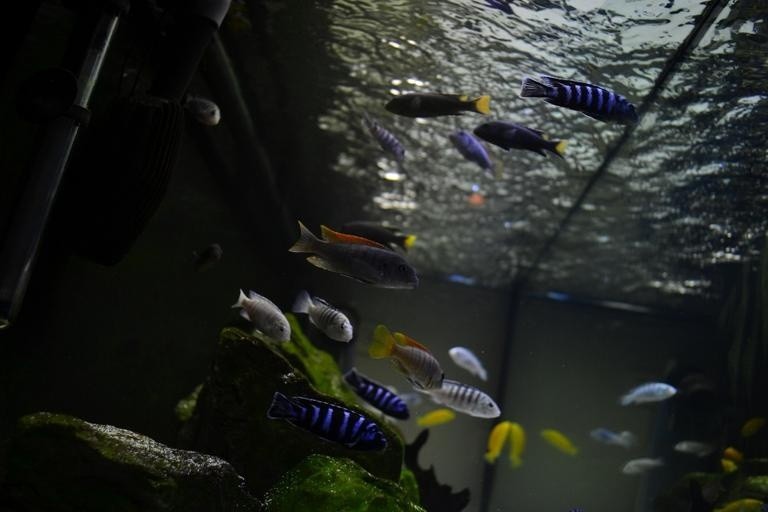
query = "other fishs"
xmin=289 ymin=216 xmax=419 ymax=290
xmin=511 ymin=372 xmax=768 ymax=511
xmin=231 ymin=288 xmax=511 ymax=463
